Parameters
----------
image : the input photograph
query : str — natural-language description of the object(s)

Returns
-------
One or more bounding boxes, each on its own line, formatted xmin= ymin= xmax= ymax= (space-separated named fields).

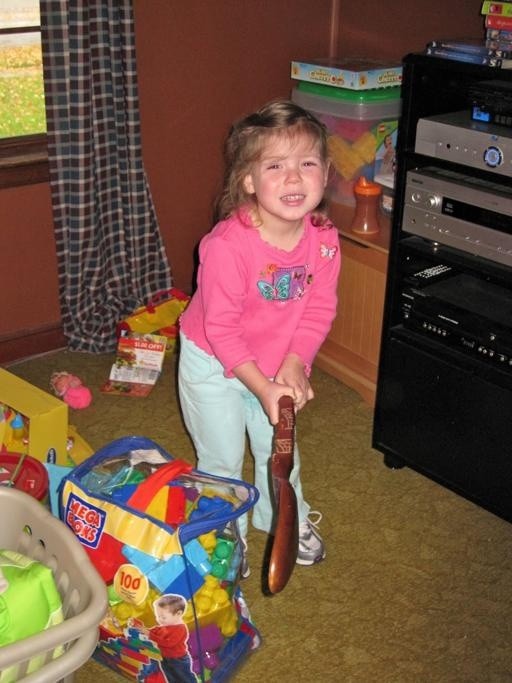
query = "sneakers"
xmin=296 ymin=511 xmax=326 ymax=565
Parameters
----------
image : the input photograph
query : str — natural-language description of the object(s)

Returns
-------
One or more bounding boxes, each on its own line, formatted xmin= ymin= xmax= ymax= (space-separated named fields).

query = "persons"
xmin=178 ymin=99 xmax=342 ymax=578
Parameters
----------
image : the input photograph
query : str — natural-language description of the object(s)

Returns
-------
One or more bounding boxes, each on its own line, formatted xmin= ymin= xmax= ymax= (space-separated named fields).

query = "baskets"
xmin=0 ymin=486 xmax=108 ymax=683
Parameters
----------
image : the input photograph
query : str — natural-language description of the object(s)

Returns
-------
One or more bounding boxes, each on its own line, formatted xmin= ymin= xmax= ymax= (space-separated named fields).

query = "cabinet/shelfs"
xmin=369 ymin=51 xmax=512 ymax=524
xmin=279 ymin=247 xmax=391 ymax=401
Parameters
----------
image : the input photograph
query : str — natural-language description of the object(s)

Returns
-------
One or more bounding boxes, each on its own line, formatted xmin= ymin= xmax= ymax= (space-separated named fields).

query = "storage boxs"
xmin=288 ymin=84 xmax=400 ymax=210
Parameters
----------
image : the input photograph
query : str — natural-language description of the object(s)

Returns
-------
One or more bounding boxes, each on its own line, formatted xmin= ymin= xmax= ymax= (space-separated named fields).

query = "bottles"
xmin=352 ymin=175 xmax=383 ymax=235
